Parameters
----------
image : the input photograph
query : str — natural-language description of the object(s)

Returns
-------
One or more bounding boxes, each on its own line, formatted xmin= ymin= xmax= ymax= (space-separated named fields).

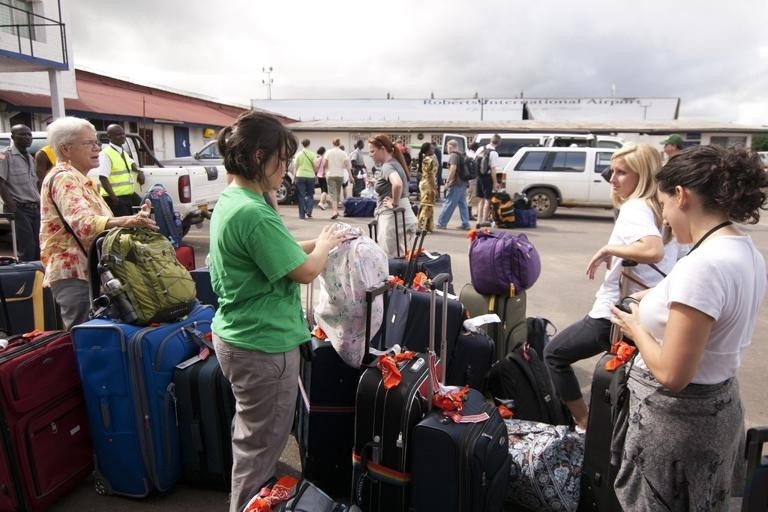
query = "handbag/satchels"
xmin=314 ymin=176 xmax=319 ymax=186
xmin=490 ymin=192 xmax=536 ymax=229
xmin=342 ymin=197 xmax=377 ymax=217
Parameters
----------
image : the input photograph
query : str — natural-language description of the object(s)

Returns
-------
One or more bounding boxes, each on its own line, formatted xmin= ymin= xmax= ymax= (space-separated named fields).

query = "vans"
xmin=441 ymin=134 xmax=626 ymax=194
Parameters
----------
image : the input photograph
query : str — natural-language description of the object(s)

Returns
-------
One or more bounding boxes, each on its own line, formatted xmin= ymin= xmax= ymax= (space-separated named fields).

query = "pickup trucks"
xmin=160 ymin=140 xmax=320 ymax=204
xmin=0 ymin=132 xmax=226 ymax=240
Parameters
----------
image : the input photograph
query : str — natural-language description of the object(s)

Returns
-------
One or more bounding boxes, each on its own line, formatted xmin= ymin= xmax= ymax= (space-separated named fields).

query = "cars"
xmin=750 ymin=151 xmax=768 ymax=177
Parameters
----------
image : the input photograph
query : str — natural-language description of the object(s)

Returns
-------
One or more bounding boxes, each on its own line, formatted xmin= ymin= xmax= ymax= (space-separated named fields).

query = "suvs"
xmin=502 ymin=147 xmax=619 ymax=218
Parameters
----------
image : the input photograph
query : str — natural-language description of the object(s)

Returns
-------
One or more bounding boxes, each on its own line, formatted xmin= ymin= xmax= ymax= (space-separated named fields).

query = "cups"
xmin=99 ymin=271 xmax=137 ymax=323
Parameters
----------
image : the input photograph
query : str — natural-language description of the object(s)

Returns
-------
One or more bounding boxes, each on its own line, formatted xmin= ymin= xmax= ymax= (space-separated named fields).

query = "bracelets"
xmin=392 ymin=203 xmax=399 ymax=208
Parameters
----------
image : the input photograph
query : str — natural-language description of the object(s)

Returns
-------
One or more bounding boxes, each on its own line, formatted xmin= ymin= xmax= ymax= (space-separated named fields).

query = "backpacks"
xmin=448 ymin=145 xmax=497 ymax=181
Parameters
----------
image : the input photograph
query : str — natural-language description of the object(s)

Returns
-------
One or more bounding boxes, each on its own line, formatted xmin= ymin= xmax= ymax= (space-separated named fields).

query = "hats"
xmin=660 ymin=133 xmax=682 ymax=147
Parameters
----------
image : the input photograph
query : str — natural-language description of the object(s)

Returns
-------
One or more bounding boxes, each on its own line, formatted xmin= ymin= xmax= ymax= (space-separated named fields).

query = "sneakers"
xmin=318 ymin=202 xmax=325 ymax=210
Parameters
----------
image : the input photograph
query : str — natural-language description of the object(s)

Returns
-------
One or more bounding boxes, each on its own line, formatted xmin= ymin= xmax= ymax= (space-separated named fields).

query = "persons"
xmin=38 ymin=116 xmax=161 ymax=333
xmin=292 ymin=138 xmax=367 ymax=220
xmin=542 ymin=142 xmax=692 ymax=436
xmin=659 ymin=134 xmax=684 ymax=158
xmin=97 ymin=123 xmax=145 ymax=217
xmin=394 ymin=133 xmax=502 ymax=235
xmin=609 ymin=142 xmax=767 ymax=512
xmin=0 ymin=124 xmax=40 ymax=262
xmin=367 ymin=134 xmax=418 ymax=258
xmin=208 ymin=109 xmax=351 ymax=512
xmin=35 ymin=143 xmax=58 ymax=194
